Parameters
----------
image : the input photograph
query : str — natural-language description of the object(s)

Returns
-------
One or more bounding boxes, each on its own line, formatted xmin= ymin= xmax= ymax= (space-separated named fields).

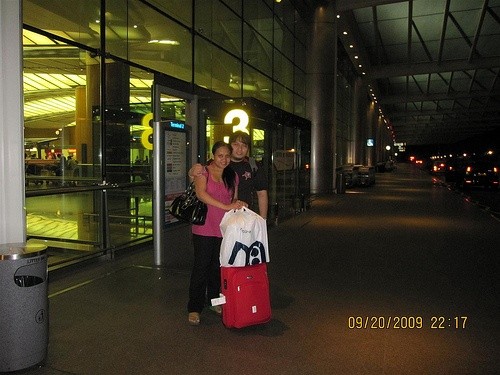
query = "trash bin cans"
xmin=0 ymin=240 xmax=51 ymax=372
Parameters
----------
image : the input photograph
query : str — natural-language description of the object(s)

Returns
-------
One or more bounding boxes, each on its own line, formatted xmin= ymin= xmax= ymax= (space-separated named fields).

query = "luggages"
xmin=221 ymin=263 xmax=272 ymax=328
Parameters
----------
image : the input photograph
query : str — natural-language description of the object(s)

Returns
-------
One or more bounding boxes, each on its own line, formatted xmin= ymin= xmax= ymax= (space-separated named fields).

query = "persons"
xmin=407 ymin=158 xmax=412 ymax=168
xmin=25 ymin=152 xmax=78 ymax=187
xmin=188 ymin=131 xmax=268 ymax=220
xmin=187 ymin=141 xmax=248 ymax=325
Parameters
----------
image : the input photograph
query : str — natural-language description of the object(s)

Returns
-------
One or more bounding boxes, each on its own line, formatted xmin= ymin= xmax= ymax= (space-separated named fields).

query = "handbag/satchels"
xmin=169 ymin=166 xmax=209 ymax=225
xmin=219 ymin=206 xmax=269 ymax=267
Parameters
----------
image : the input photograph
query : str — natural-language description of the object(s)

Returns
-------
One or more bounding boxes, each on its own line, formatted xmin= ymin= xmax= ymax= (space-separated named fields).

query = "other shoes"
xmin=209 ymin=305 xmax=222 ymax=314
xmin=189 ymin=312 xmax=200 ymax=325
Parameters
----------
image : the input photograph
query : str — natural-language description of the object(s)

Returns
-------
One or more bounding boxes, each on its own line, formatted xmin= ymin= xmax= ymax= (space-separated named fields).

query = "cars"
xmin=434 ymin=156 xmax=500 ymax=194
